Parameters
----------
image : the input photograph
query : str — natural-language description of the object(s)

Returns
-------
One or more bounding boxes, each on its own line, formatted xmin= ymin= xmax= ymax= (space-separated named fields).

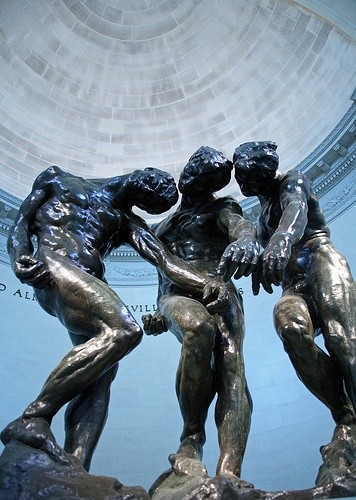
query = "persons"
xmin=141 ymin=145 xmax=258 ymax=489
xmin=0 ymin=165 xmax=232 ymax=473
xmin=232 ymin=139 xmax=356 ymax=452
